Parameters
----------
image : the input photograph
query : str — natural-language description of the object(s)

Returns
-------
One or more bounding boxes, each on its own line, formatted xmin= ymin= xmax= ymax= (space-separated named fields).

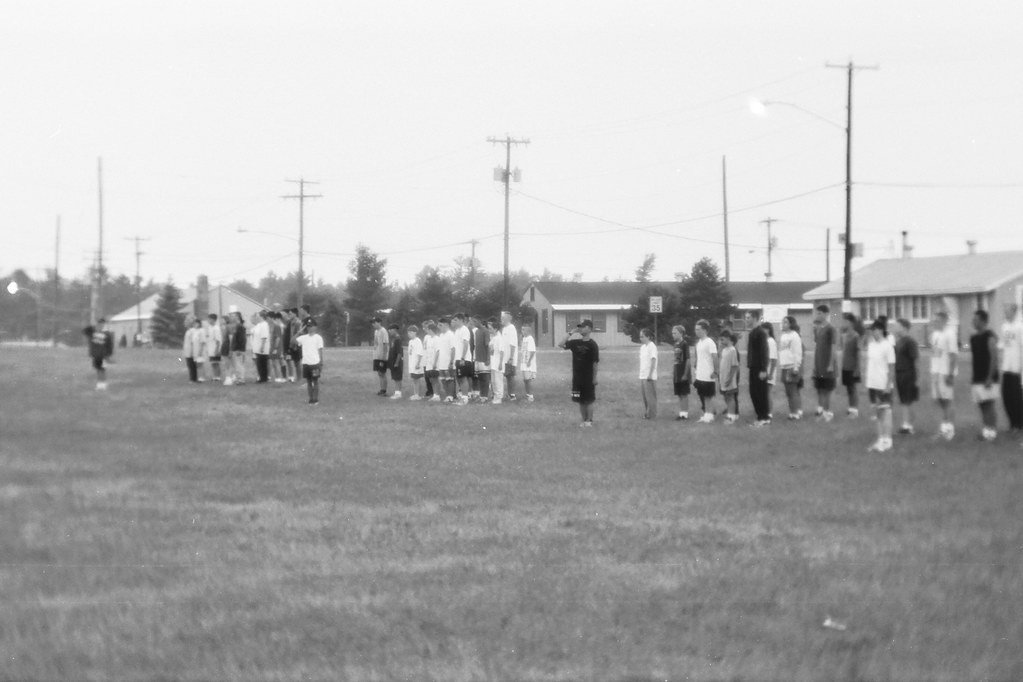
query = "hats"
xmin=387 ymin=323 xmax=399 ymax=330
xmin=370 ymin=317 xmax=380 ymax=323
xmin=306 ymin=319 xmax=316 ymax=326
xmin=865 ymin=320 xmax=884 ymax=328
xmin=577 ymin=319 xmax=593 ymax=328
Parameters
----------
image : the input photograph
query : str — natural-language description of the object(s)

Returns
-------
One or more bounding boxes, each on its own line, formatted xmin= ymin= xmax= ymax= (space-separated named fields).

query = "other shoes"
xmin=455 ymin=391 xmax=534 ymax=405
xmin=580 ymin=408 xmax=998 ymax=454
xmin=308 ymin=399 xmax=319 ymax=406
xmin=391 ymin=393 xmax=402 ymax=400
xmin=377 ymin=389 xmax=386 ymax=395
xmin=410 ymin=394 xmax=421 ymax=400
xmin=190 ymin=375 xmax=299 ymax=385
xmin=428 ymin=394 xmax=441 ymax=401
xmin=444 ymin=395 xmax=454 ymax=402
xmin=95 ymin=381 xmax=107 ymax=390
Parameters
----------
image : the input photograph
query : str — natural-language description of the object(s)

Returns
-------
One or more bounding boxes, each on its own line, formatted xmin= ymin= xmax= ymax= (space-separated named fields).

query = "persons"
xmin=559 ymin=320 xmax=599 ymax=426
xmin=80 ymin=313 xmax=116 ymax=391
xmin=927 ymin=310 xmax=960 ymax=443
xmin=866 ymin=320 xmax=895 ymax=452
xmin=997 ymin=301 xmax=1022 ymax=433
xmin=291 ymin=320 xmax=322 ymax=404
xmin=639 ymin=328 xmax=658 ymax=419
xmin=368 ymin=310 xmax=538 ymax=405
xmin=668 ymin=305 xmax=918 ymax=436
xmin=968 ymin=308 xmax=996 ymax=444
xmin=184 ymin=306 xmax=308 ymax=386
